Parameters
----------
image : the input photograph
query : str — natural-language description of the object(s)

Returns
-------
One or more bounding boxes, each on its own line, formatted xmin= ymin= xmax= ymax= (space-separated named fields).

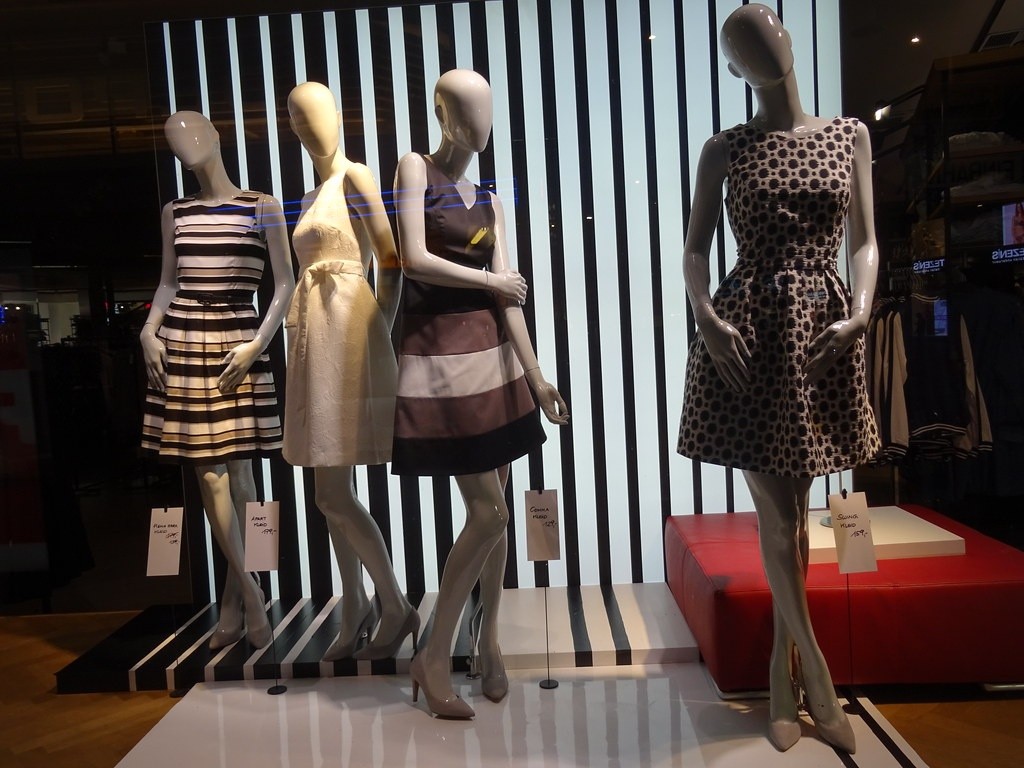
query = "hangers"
xmin=885 ymin=258 xmax=971 ymax=298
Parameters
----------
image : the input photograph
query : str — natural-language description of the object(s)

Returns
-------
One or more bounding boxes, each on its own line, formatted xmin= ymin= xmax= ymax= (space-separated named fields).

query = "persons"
xmin=675 ymin=3 xmax=878 ymax=756
xmin=1012 ymin=202 xmax=1024 ymax=245
xmin=391 ymin=67 xmax=572 ymax=720
xmin=138 ymin=109 xmax=298 ymax=652
xmin=279 ymin=79 xmax=421 ymax=663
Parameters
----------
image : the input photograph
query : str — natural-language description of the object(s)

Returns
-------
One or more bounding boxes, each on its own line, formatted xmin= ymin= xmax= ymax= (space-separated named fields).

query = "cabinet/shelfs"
xmin=900 ymin=45 xmax=1024 ymax=300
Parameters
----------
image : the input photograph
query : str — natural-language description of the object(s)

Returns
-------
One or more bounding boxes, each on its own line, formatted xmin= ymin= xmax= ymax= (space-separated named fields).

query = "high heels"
xmin=797 ymin=662 xmax=856 ymax=755
xmin=321 ymin=603 xmax=377 ymax=662
xmin=242 ymin=586 xmax=272 ymax=649
xmin=478 ymin=640 xmax=508 ymax=701
xmin=769 ymin=662 xmax=802 ymax=751
xmin=351 ymin=606 xmax=421 ymax=662
xmin=208 ymin=587 xmax=245 ymax=648
xmin=410 ymin=654 xmax=476 ymax=719
